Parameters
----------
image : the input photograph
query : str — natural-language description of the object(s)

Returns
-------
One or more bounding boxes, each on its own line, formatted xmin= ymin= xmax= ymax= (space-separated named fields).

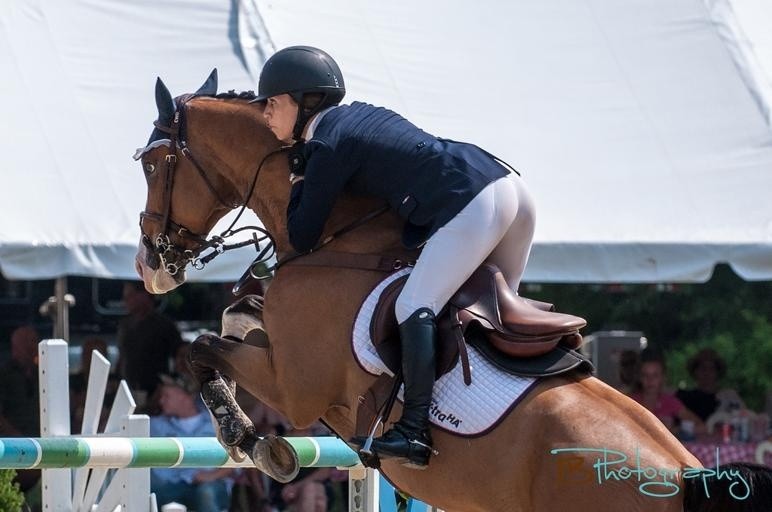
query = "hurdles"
xmin=0 ymin=435 xmax=380 ymax=512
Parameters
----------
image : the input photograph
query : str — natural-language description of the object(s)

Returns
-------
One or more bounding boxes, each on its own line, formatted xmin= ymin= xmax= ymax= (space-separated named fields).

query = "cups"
xmin=680 ymin=419 xmax=696 ymax=440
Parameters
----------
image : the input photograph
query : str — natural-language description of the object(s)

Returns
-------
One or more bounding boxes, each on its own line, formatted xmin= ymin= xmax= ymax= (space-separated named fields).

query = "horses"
xmin=132 ymin=67 xmax=772 ymax=512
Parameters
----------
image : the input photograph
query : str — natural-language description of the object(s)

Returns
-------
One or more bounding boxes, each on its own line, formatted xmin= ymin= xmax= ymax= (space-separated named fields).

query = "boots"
xmin=346 ymin=306 xmax=436 ymax=472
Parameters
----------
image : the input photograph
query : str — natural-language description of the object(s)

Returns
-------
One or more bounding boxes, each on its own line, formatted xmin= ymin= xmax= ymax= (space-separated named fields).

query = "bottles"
xmin=719 ymin=408 xmax=768 ymax=443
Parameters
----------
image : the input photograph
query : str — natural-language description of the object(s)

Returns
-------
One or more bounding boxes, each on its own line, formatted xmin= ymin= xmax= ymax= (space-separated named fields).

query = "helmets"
xmin=247 ymin=45 xmax=346 ymax=105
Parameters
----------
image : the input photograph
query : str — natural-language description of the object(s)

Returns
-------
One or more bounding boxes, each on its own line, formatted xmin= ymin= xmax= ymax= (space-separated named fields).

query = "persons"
xmin=0 ymin=282 xmax=348 ymax=512
xmin=247 ymin=45 xmax=535 ymax=470
xmin=601 ymin=349 xmax=749 ymax=440
